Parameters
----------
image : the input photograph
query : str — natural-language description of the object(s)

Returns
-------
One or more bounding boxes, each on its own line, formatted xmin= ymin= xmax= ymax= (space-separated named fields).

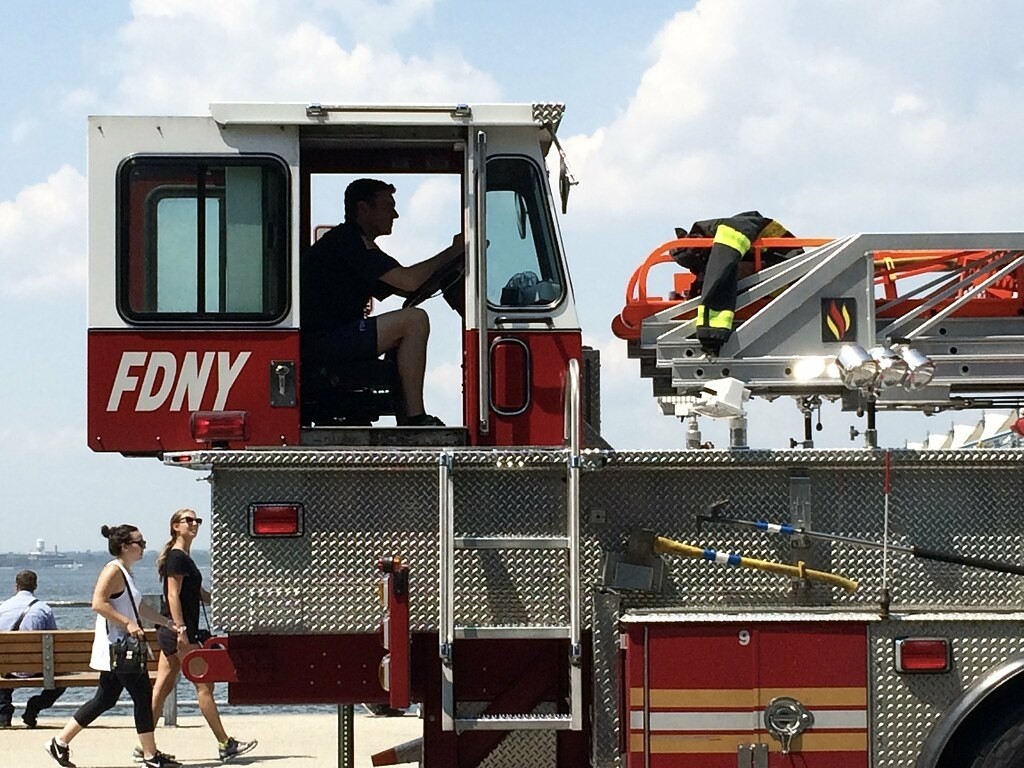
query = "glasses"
xmin=177 ymin=516 xmax=202 ymax=526
xmin=126 ymin=540 xmax=146 ymax=549
xmin=374 ymin=185 xmax=395 ymax=196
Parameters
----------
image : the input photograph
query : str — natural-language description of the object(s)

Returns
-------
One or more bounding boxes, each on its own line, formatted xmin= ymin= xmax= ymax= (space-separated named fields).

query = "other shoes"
xmin=0 ymin=719 xmax=11 ymax=729
xmin=21 ymin=712 xmax=37 ymax=727
xmin=399 ymin=413 xmax=446 ymax=426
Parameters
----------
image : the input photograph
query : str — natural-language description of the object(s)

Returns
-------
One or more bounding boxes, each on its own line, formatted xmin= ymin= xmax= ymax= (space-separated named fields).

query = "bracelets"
xmin=166 ymin=620 xmax=172 ymax=627
xmin=180 ymin=625 xmax=187 ymax=631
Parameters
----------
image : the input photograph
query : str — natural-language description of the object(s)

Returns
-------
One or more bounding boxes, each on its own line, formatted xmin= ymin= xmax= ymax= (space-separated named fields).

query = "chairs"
xmin=302 ymin=354 xmax=407 ymax=421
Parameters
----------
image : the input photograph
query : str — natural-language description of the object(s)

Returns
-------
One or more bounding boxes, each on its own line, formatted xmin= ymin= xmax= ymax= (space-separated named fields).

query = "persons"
xmin=0 ymin=569 xmax=67 ymax=728
xmin=130 ymin=508 xmax=258 ymax=764
xmin=45 ymin=524 xmax=186 ymax=768
xmin=303 ymin=179 xmax=463 ymax=426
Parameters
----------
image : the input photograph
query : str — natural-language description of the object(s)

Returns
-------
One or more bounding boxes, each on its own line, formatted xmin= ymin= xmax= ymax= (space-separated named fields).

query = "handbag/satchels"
xmin=198 ymin=628 xmax=222 ymax=650
xmin=109 ymin=633 xmax=147 ymax=672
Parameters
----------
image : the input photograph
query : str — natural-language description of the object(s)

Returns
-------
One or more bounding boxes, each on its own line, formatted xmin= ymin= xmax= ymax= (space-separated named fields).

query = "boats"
xmin=0 ymin=545 xmax=83 ymax=570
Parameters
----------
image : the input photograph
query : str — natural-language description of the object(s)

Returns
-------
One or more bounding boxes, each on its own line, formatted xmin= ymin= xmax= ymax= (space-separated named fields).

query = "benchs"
xmin=0 ymin=629 xmax=181 ymax=728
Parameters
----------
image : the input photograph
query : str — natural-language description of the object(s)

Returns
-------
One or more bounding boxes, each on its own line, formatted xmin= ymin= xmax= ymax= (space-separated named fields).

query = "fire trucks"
xmin=84 ymin=105 xmax=1024 ymax=768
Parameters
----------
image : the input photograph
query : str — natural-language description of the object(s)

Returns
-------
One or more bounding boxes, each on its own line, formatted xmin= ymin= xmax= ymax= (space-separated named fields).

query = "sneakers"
xmin=141 ymin=750 xmax=182 ymax=768
xmin=44 ymin=737 xmax=76 ymax=768
xmin=132 ymin=748 xmax=175 ymax=762
xmin=219 ymin=737 xmax=258 ymax=763
